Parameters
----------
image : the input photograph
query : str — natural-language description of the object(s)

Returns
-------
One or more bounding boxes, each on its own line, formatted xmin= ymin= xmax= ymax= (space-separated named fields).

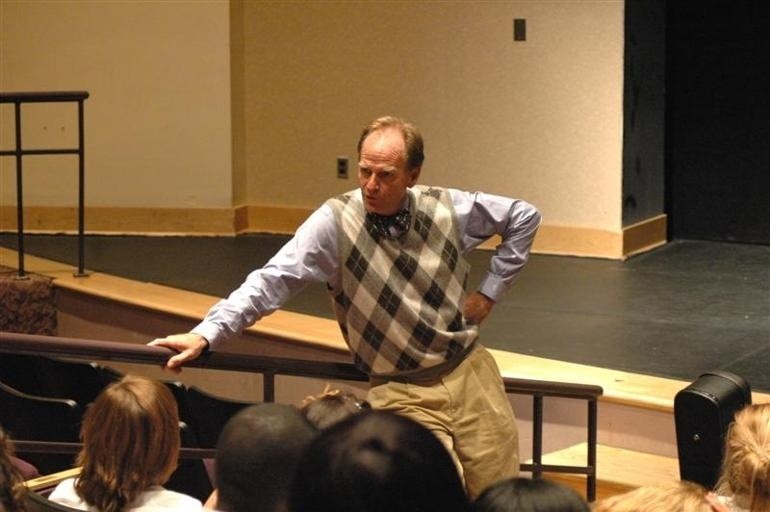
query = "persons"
xmin=198 ymin=394 xmax=473 ymax=511
xmin=146 ymin=116 xmax=542 ymax=503
xmin=45 ymin=373 xmax=203 ymax=511
xmin=1 ymin=433 xmax=31 ymax=512
xmin=588 ymin=480 xmax=731 ymax=512
xmin=710 ymin=402 xmax=770 ymax=512
xmin=473 ymin=476 xmax=591 ymax=512
